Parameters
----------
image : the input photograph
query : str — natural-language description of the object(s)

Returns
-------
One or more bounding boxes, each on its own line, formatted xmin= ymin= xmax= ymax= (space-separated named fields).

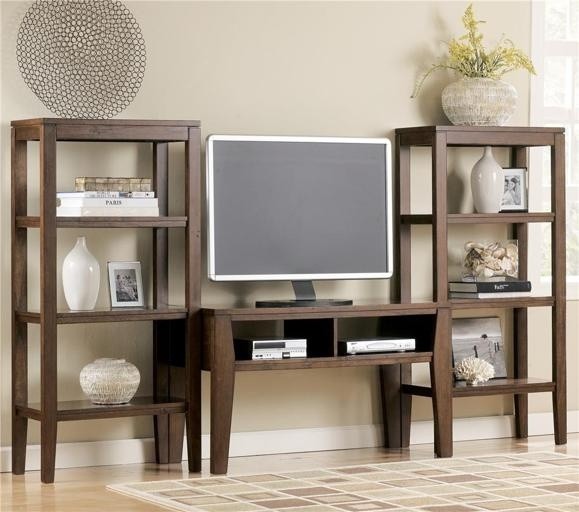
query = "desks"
xmin=170 ymin=302 xmax=451 ymax=473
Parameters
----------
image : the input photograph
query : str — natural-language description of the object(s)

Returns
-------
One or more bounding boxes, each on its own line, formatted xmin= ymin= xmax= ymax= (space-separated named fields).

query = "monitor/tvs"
xmin=204 ymin=132 xmax=395 ymax=306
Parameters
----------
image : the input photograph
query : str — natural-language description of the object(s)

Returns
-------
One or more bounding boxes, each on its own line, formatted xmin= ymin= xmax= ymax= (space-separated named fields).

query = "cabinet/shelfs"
xmin=8 ymin=118 xmax=201 ymax=485
xmin=396 ymin=124 xmax=576 ymax=457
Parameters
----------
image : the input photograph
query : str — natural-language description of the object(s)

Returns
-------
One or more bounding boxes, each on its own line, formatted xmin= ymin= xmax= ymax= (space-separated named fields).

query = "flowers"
xmin=454 ymin=356 xmax=494 ymax=385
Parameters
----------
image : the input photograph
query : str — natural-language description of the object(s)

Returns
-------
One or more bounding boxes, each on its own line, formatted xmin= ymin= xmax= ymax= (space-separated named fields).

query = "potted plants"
xmin=408 ymin=2 xmax=536 ymax=124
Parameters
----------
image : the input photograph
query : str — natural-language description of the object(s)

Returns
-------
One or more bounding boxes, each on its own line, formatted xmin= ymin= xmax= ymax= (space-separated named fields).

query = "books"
xmin=449 ymin=291 xmax=531 ymax=299
xmin=56 ymin=191 xmax=160 ymax=217
xmin=448 ymin=280 xmax=531 ymax=293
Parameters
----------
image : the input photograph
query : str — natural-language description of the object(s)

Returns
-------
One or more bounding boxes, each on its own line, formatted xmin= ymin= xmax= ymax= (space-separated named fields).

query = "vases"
xmin=62 ymin=235 xmax=100 ymax=312
xmin=79 ymin=357 xmax=141 ymax=405
xmin=470 ymin=145 xmax=503 ymax=213
xmin=465 ymin=376 xmax=478 ymax=384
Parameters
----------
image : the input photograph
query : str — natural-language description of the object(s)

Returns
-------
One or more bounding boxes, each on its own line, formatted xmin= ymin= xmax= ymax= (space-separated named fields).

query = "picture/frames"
xmin=451 ymin=315 xmax=508 ymax=380
xmin=107 ymin=261 xmax=145 ymax=307
xmin=500 ymin=166 xmax=528 ymax=213
